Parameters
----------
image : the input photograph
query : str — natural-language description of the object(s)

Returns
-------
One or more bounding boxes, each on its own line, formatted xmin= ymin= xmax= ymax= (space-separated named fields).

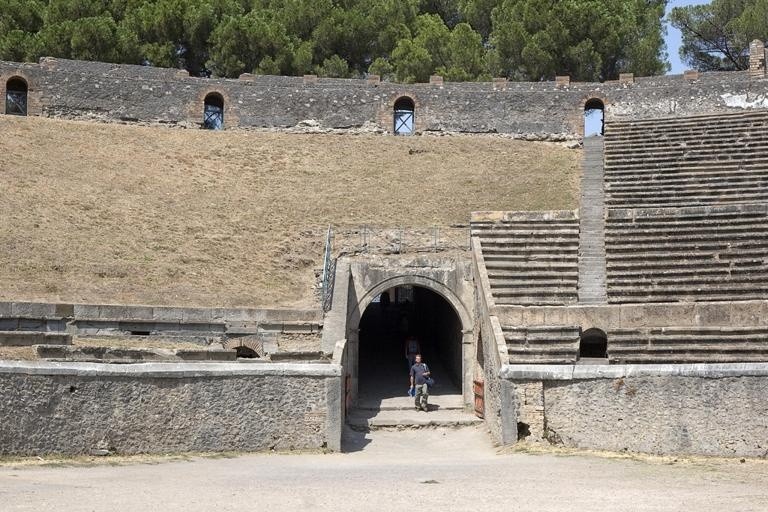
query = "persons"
xmin=405 ymin=333 xmax=422 ymax=372
xmin=407 ymin=353 xmax=431 ymax=412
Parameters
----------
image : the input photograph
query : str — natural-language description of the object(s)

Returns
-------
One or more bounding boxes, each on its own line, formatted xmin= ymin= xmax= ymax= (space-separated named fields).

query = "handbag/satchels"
xmin=427 ymin=378 xmax=434 ymax=387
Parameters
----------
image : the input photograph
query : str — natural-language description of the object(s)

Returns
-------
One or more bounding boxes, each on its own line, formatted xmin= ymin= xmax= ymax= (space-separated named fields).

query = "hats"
xmin=408 ymin=388 xmax=415 ymax=397
